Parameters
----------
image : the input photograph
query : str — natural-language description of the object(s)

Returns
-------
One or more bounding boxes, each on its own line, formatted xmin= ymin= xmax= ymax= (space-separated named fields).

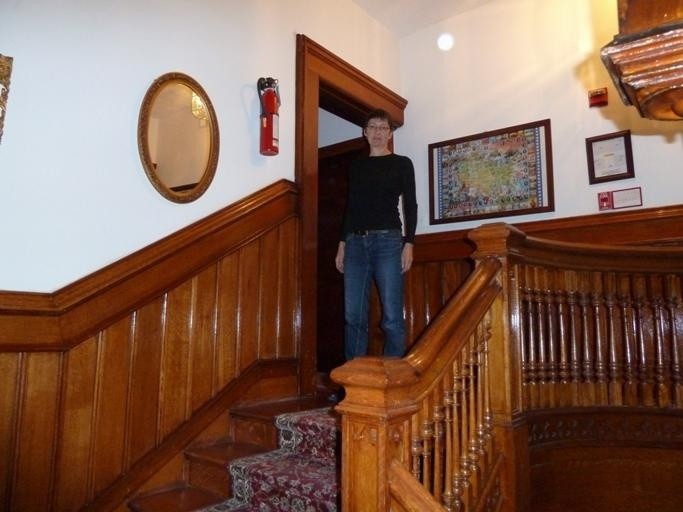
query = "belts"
xmin=348 ymin=227 xmax=404 ymax=238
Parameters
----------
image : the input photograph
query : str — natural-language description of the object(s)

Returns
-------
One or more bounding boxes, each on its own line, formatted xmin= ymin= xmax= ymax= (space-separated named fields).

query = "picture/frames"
xmin=583 ymin=128 xmax=636 ymax=186
xmin=426 ymin=117 xmax=555 ymax=226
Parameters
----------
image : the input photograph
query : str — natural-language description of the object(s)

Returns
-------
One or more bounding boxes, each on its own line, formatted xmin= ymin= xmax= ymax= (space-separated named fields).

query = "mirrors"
xmin=136 ymin=71 xmax=220 ymax=205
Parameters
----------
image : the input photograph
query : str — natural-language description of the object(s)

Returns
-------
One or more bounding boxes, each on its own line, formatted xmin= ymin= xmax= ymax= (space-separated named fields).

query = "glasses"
xmin=365 ymin=125 xmax=391 ymax=129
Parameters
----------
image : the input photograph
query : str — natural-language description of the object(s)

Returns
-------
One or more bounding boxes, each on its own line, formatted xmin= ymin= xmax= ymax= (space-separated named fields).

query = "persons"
xmin=326 ymin=107 xmax=419 ymax=402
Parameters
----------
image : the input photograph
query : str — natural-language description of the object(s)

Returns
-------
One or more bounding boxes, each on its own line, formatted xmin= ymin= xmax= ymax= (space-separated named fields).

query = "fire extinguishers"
xmin=256 ymin=77 xmax=280 ymax=156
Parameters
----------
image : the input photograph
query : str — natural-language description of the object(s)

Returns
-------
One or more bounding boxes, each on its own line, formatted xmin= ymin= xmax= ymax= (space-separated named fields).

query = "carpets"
xmin=191 ymin=405 xmax=340 ymax=512
